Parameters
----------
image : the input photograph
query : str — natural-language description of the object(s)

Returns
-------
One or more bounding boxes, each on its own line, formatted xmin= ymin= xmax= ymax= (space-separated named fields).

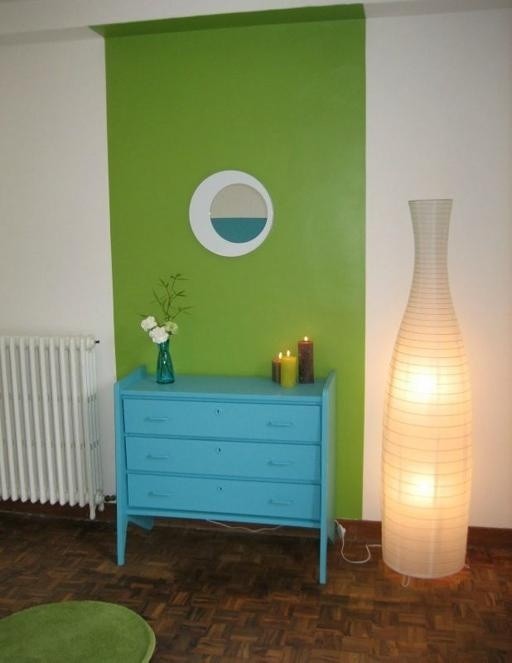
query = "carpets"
xmin=0 ymin=598 xmax=166 ymax=662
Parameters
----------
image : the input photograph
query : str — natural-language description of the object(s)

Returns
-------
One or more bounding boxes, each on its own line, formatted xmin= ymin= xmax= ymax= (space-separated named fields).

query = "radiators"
xmin=0 ymin=332 xmax=116 ymax=519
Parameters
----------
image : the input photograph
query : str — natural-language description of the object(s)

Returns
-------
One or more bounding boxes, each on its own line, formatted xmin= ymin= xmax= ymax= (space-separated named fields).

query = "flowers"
xmin=137 ymin=269 xmax=193 ymax=347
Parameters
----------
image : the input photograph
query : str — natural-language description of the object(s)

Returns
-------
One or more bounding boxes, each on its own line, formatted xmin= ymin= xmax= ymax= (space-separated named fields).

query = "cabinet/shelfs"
xmin=108 ymin=368 xmax=343 ymax=588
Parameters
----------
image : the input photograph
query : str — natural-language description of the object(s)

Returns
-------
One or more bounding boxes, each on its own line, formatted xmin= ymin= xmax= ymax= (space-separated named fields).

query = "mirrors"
xmin=185 ymin=168 xmax=277 ymax=259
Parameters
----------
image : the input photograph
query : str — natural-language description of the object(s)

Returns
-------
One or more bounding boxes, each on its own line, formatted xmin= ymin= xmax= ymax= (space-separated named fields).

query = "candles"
xmin=271 ymin=353 xmax=285 ymax=382
xmin=279 ymin=349 xmax=299 ymax=388
xmin=297 ymin=334 xmax=315 ymax=384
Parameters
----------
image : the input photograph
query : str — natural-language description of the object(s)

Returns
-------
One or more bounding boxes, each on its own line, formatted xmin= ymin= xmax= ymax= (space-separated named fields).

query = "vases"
xmin=150 ymin=337 xmax=179 ymax=386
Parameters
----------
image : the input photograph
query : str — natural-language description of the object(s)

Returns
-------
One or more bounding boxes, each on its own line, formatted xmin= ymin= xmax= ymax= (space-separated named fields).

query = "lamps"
xmin=335 ymin=195 xmax=478 ymax=581
